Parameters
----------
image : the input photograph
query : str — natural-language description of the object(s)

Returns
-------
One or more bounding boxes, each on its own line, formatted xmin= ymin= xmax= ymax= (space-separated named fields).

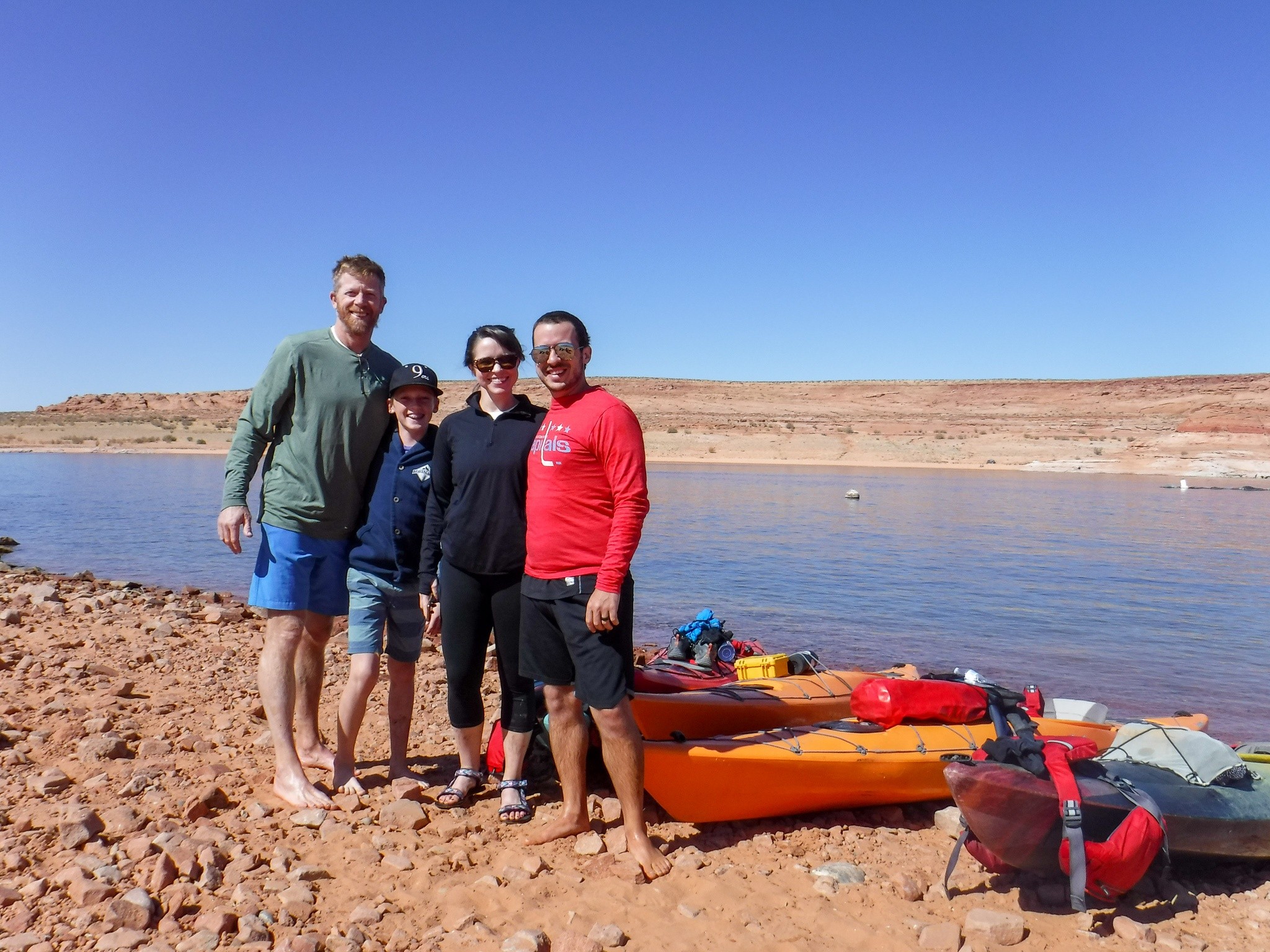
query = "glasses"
xmin=530 ymin=342 xmax=586 ymax=365
xmin=470 ymin=354 xmax=519 ymax=372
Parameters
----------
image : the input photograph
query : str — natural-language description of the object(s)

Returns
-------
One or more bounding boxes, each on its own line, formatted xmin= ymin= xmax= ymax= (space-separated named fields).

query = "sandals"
xmin=498 ymin=779 xmax=532 ymax=826
xmin=434 ymin=767 xmax=485 ymax=807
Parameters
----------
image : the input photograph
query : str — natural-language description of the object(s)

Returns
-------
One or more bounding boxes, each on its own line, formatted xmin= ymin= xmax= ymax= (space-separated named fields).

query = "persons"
xmin=519 ymin=309 xmax=674 ymax=882
xmin=331 ymin=361 xmax=445 ymax=796
xmin=416 ymin=324 xmax=550 ymax=825
xmin=213 ymin=255 xmax=406 ymax=812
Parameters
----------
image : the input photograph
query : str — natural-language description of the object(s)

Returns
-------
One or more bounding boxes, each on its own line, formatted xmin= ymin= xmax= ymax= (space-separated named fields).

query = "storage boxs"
xmin=733 ymin=653 xmax=789 ymax=681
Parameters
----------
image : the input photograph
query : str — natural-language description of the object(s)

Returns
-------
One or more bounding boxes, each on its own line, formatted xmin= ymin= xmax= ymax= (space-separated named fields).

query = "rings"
xmin=601 ymin=615 xmax=609 ymax=621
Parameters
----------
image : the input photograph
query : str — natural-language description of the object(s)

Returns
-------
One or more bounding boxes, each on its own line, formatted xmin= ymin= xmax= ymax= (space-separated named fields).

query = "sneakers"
xmin=668 ymin=635 xmax=688 ymax=659
xmin=693 ymin=641 xmax=717 ymax=667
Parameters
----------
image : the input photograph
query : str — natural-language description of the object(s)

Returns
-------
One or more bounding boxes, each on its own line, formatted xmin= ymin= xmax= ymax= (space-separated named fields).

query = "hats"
xmin=390 ymin=364 xmax=445 ymax=397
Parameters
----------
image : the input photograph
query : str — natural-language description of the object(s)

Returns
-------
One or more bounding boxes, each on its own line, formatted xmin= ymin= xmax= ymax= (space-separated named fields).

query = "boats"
xmin=632 ymin=638 xmax=765 ymax=693
xmin=640 ymin=712 xmax=1207 ymax=822
xmin=627 ymin=664 xmax=924 ymax=742
xmin=943 ymin=743 xmax=1270 ymax=878
